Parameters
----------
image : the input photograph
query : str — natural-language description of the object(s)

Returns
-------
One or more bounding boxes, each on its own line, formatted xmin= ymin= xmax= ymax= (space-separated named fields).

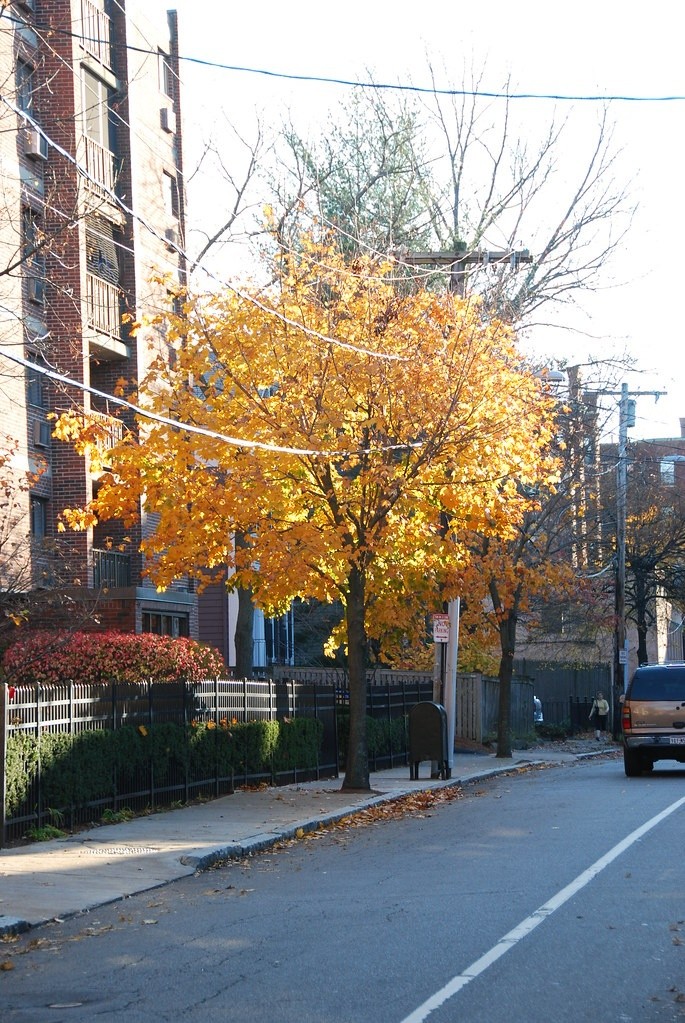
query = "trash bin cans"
xmin=408 ymin=700 xmax=447 ymax=780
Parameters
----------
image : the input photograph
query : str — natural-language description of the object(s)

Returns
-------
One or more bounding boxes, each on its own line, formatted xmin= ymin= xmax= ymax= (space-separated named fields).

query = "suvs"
xmin=619 ymin=660 xmax=685 ymax=777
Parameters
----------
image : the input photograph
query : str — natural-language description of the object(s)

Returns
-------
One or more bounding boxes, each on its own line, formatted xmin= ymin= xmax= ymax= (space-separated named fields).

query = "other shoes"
xmin=603 ymin=737 xmax=607 ymax=741
xmin=596 ymin=737 xmax=601 ymax=741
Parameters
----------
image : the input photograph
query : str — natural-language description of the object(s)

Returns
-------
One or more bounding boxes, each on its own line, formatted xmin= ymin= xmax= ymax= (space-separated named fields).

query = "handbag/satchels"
xmin=593 ymin=699 xmax=599 ymax=719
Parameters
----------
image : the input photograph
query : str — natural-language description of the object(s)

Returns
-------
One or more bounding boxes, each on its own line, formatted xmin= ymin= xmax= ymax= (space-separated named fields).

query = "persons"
xmin=589 ymin=692 xmax=609 ymax=741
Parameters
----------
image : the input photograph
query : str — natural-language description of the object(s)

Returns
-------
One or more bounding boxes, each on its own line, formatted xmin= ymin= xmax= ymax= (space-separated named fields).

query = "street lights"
xmin=612 ymin=454 xmax=685 ymax=743
